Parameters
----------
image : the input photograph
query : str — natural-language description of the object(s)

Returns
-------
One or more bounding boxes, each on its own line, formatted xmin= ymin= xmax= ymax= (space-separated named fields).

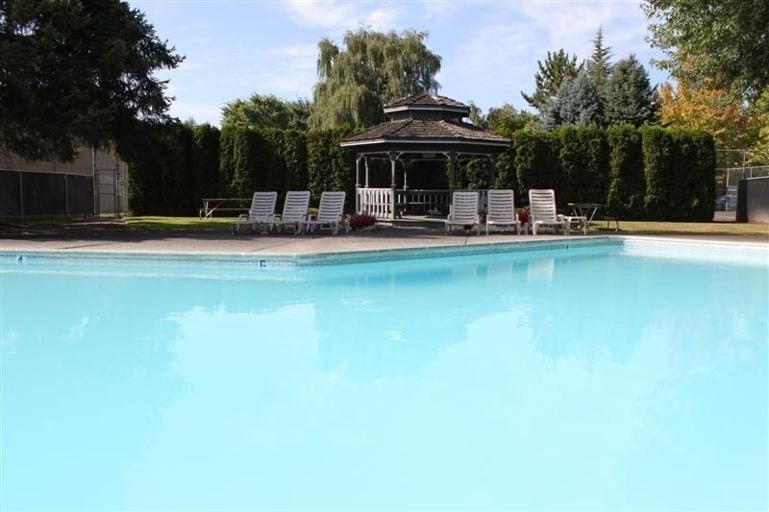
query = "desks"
xmin=567 ymin=203 xmax=602 ymax=232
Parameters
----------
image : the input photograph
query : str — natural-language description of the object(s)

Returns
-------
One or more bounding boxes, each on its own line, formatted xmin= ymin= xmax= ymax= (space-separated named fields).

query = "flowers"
xmin=349 ymin=213 xmax=376 ymax=229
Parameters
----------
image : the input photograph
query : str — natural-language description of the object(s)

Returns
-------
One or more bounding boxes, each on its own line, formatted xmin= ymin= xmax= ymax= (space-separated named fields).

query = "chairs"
xmin=232 ymin=190 xmax=347 ymax=237
xmin=443 ymin=188 xmax=568 ymax=237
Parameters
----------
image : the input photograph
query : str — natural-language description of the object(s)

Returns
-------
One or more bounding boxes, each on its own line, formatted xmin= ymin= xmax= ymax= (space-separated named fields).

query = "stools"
xmin=564 ymin=213 xmax=624 ymax=235
